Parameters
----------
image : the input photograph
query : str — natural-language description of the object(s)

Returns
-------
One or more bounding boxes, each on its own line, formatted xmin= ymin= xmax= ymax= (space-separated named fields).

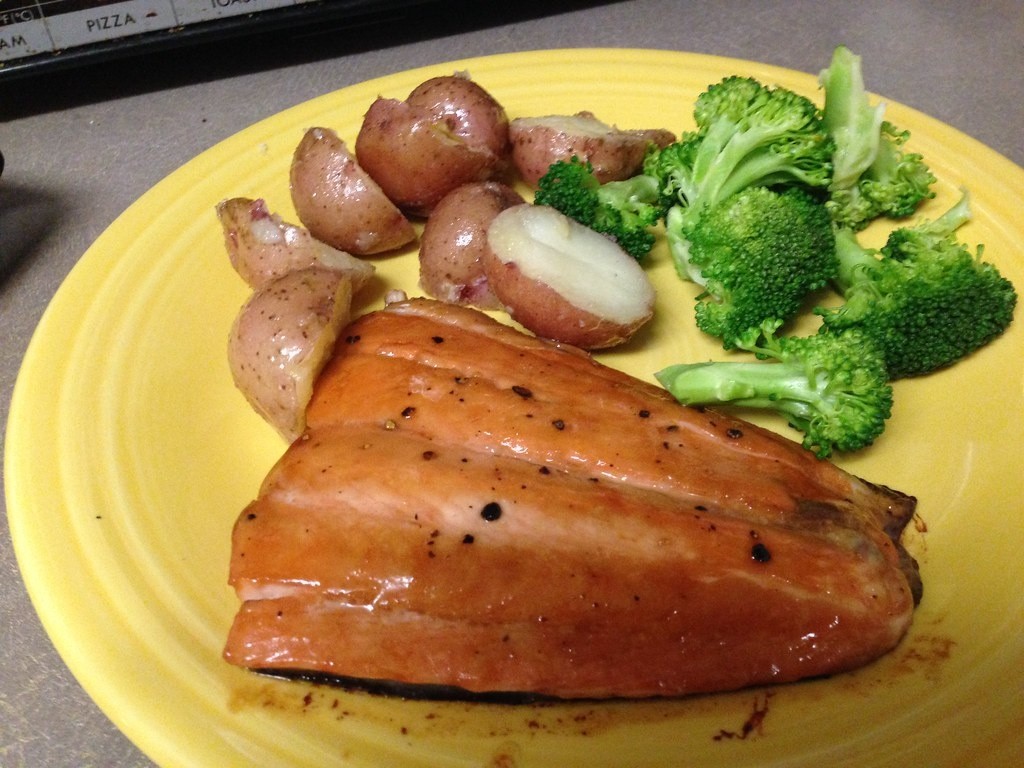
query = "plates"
xmin=4 ymin=46 xmax=1024 ymax=768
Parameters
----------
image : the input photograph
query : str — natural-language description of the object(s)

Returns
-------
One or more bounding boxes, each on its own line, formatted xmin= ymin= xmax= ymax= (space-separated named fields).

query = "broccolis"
xmin=532 ymin=45 xmax=1018 ymax=460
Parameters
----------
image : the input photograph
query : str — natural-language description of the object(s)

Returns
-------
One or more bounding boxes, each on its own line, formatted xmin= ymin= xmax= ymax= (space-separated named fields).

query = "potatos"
xmin=215 ymin=74 xmax=677 ymax=435
